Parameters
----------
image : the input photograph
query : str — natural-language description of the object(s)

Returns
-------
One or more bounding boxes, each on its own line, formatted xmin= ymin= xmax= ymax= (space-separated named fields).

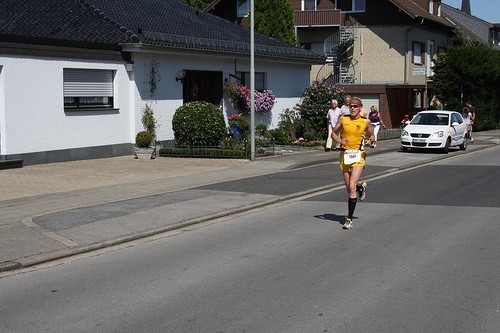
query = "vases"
xmin=232 ymin=124 xmax=244 ymax=140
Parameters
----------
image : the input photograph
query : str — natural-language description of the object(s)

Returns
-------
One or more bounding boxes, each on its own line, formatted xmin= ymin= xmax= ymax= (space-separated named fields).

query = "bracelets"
xmin=369 ymin=138 xmax=373 ymax=145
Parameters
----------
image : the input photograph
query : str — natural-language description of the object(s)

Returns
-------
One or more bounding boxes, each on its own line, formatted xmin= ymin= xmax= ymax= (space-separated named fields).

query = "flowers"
xmin=227 ymin=113 xmax=239 ymax=120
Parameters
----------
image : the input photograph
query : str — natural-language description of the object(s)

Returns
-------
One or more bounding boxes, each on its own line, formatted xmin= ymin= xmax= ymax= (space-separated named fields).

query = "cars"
xmin=400 ymin=110 xmax=468 ymax=154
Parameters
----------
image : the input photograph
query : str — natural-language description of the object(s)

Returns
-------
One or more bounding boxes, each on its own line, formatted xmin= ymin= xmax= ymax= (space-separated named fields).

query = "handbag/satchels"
xmin=343 ymin=150 xmax=361 ymax=165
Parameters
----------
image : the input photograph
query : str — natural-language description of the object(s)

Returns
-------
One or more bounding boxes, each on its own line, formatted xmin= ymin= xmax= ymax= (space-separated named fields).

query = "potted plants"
xmin=134 ymin=131 xmax=156 ymax=159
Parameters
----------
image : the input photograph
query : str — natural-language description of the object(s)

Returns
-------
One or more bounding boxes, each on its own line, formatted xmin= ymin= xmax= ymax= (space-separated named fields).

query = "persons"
xmin=462 ymin=107 xmax=472 ymax=139
xmin=466 ymin=100 xmax=475 ymax=121
xmin=401 ymin=114 xmax=410 ymax=128
xmin=331 ymin=98 xmax=375 ymax=229
xmin=325 ymin=100 xmax=341 ymax=151
xmin=341 ymin=95 xmax=352 ymax=115
xmin=359 ymin=104 xmax=368 ymax=119
xmin=368 ymin=105 xmax=387 ymax=148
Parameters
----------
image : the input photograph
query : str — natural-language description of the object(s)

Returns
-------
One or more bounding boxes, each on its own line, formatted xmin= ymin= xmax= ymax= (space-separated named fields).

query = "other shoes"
xmin=336 ymin=148 xmax=341 ymax=151
xmin=325 ymin=148 xmax=330 ymax=151
xmin=370 ymin=142 xmax=378 ymax=147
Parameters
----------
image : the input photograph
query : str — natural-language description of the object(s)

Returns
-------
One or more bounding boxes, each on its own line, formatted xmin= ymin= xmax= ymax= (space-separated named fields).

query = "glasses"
xmin=350 ymin=105 xmax=360 ymax=108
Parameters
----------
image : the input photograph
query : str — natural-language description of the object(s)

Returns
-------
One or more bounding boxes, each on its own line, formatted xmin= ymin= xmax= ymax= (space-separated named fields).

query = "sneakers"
xmin=359 ymin=182 xmax=368 ymax=201
xmin=342 ymin=218 xmax=354 ymax=229
